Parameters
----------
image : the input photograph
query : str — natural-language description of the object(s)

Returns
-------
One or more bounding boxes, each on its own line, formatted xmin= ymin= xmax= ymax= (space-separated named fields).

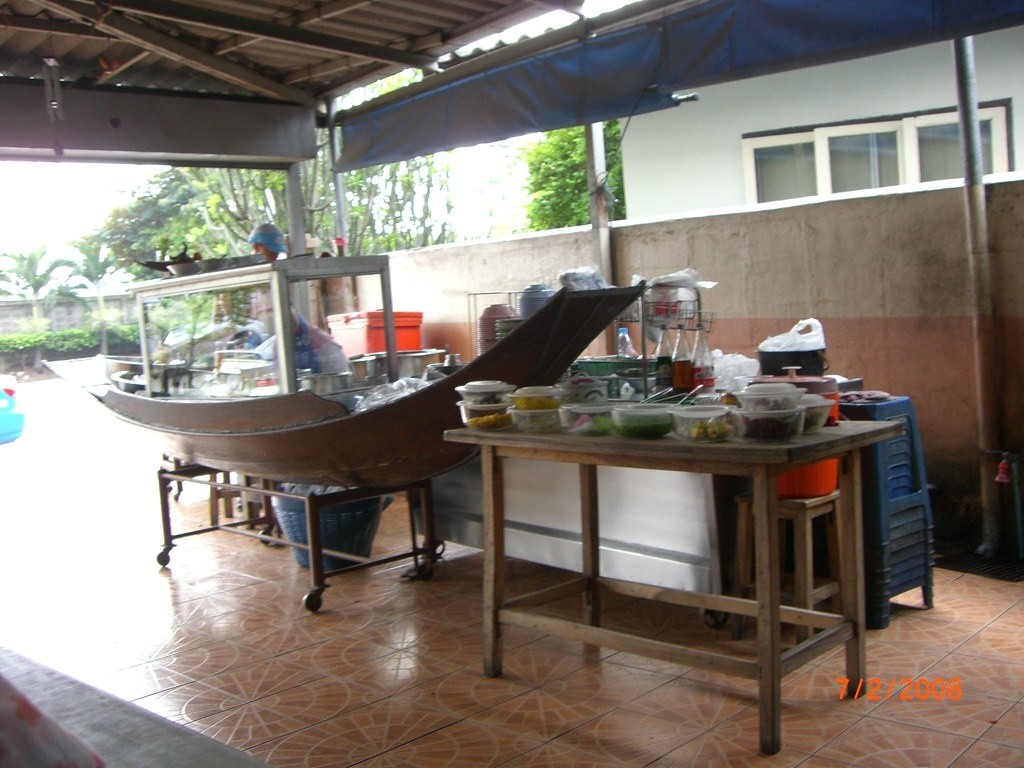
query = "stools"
xmin=736 ymin=491 xmax=836 ymax=646
xmin=836 ymin=396 xmax=937 ymax=629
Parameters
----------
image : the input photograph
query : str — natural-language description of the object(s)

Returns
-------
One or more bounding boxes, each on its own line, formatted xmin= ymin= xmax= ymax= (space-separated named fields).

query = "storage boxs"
xmin=326 ymin=310 xmax=423 ymax=356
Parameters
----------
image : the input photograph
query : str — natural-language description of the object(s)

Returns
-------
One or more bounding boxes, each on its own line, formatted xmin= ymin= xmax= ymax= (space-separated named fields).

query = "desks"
xmin=441 ymin=421 xmax=904 ymax=755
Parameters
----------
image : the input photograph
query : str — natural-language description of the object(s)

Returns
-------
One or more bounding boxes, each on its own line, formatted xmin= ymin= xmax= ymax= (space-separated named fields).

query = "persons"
xmin=236 ymin=223 xmax=288 ymax=511
xmin=288 ymin=303 xmax=349 ymax=373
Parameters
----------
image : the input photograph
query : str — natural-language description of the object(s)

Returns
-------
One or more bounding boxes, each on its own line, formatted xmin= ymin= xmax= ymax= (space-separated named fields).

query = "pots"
xmin=296 ymin=350 xmax=446 ymax=396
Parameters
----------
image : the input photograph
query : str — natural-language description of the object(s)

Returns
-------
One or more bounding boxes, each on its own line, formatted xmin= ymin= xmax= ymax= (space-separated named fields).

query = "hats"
xmin=248 ymin=223 xmax=289 ymax=254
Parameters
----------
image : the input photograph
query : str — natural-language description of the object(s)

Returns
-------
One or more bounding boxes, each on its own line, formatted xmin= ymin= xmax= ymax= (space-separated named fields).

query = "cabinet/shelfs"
xmin=471 ymin=286 xmax=715 ymax=405
xmin=134 ymin=252 xmax=438 ymax=610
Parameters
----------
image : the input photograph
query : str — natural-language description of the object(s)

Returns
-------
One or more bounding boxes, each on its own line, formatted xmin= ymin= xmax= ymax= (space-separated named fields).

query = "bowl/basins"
xmin=476 ymin=284 xmax=556 ymax=357
xmin=454 ymin=379 xmax=836 ymax=442
xmin=166 ymin=253 xmax=265 ymax=275
xmin=110 ymin=370 xmax=146 ymax=394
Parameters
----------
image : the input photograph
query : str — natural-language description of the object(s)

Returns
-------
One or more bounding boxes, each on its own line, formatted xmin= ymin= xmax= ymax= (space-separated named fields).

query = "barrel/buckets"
xmin=757 ymin=348 xmax=831 ymax=377
xmin=747 ymin=366 xmax=839 ymax=498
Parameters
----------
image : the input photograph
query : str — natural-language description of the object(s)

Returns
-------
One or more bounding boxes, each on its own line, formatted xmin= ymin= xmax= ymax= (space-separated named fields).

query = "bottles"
xmin=671 ymin=324 xmax=691 ymax=393
xmin=691 ymin=322 xmax=717 ymax=395
xmin=618 ymin=328 xmax=633 ymax=357
xmin=654 ymin=324 xmax=673 ymax=393
